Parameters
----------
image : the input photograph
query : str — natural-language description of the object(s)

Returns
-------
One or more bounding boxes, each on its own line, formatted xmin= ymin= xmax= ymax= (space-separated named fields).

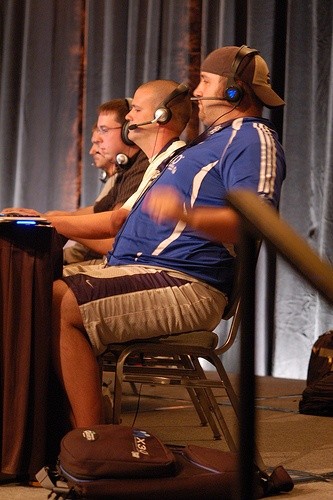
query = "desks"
xmin=0 ymin=227 xmax=66 ymax=481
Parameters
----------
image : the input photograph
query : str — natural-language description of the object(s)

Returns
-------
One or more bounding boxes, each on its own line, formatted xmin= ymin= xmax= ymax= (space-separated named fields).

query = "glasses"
xmin=96 ymin=126 xmax=122 ymax=134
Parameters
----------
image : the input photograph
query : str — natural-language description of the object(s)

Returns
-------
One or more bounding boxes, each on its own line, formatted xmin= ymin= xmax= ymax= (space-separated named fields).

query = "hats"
xmin=201 ymin=46 xmax=286 ymax=110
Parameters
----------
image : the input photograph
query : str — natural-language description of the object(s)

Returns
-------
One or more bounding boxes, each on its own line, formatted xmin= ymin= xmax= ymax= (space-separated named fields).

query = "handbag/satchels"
xmin=303 ymin=330 xmax=333 ymax=385
xmin=298 ymin=370 xmax=333 ymax=418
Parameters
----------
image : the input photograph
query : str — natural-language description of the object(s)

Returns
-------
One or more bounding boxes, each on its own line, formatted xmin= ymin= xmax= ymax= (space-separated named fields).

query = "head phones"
xmin=223 ymin=44 xmax=259 ymax=104
xmin=121 ymin=96 xmax=135 ymax=144
xmin=100 ymin=149 xmax=144 ymax=182
xmin=155 ymin=82 xmax=190 ymax=123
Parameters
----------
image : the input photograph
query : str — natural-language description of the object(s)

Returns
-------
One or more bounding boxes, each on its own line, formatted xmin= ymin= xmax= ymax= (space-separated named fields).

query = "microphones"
xmin=96 ymin=151 xmax=116 ymax=164
xmin=129 ymin=112 xmax=164 ymax=129
xmin=191 ymin=97 xmax=231 ymax=102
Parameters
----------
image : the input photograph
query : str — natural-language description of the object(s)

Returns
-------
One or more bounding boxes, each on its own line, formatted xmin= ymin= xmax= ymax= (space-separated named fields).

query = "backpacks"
xmin=34 ymin=425 xmax=295 ymax=500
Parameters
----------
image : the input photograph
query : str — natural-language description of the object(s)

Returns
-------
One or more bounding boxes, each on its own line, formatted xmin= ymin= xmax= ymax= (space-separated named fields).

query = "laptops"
xmin=0 ymin=214 xmax=47 ymax=225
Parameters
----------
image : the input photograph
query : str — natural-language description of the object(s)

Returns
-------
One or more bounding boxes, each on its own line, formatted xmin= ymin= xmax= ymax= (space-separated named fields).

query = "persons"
xmin=42 ymin=97 xmax=149 ymax=265
xmin=0 ymin=80 xmax=191 ymax=274
xmin=49 ymin=45 xmax=286 ymax=428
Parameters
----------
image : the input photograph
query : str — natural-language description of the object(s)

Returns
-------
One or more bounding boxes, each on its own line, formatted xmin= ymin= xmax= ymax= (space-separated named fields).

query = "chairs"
xmin=101 ymin=236 xmax=266 ymax=470
